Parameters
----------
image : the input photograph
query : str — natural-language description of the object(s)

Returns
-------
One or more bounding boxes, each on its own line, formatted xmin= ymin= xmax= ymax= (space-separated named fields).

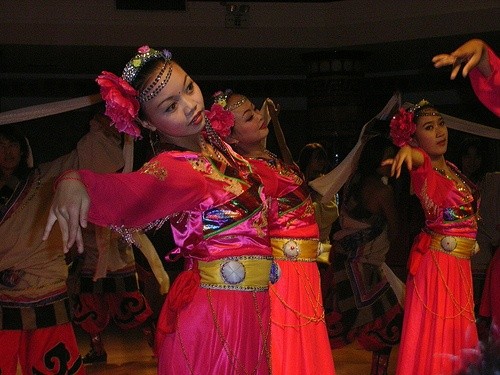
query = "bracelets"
xmin=56 ymin=175 xmax=83 ymax=185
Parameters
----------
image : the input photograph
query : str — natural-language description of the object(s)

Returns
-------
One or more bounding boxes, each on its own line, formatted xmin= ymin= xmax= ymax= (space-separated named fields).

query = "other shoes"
xmin=82 ymin=351 xmax=106 ymax=363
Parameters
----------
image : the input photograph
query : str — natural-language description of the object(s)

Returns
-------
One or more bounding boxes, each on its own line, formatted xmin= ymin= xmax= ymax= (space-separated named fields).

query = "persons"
xmin=0 ymin=101 xmax=157 ymax=375
xmin=43 ymin=43 xmax=280 ymax=375
xmin=432 ymin=38 xmax=500 ymax=375
xmin=213 ymin=88 xmax=337 ymax=375
xmin=268 ymin=99 xmax=409 ymax=343
xmin=380 ymin=100 xmax=484 ymax=375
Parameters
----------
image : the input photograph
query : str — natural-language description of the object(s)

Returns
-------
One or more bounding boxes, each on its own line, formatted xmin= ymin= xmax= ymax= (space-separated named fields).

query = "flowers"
xmin=96 ymin=72 xmax=142 ymax=141
xmin=390 ymin=108 xmax=418 ymax=148
xmin=204 ymin=103 xmax=236 ymax=140
